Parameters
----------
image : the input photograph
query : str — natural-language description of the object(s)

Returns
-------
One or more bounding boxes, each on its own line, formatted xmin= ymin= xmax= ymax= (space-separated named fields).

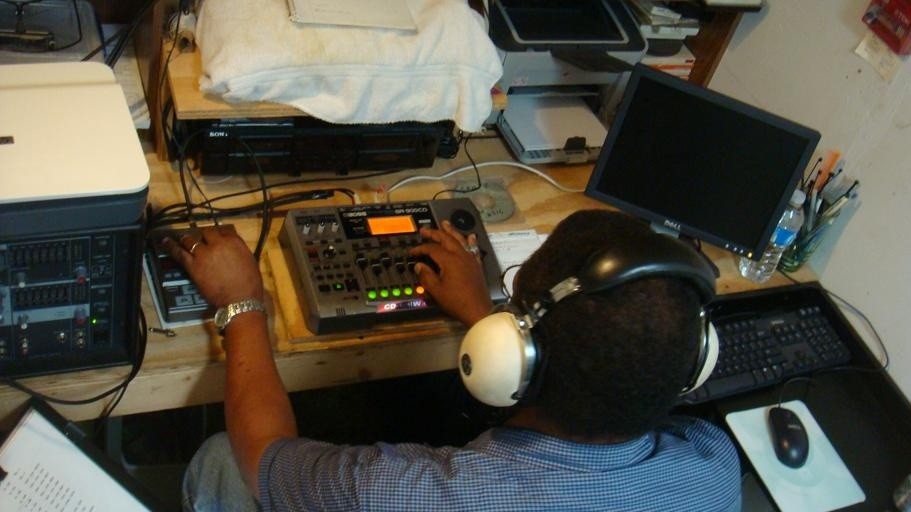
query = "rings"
xmin=468 ymin=244 xmax=480 ymax=256
xmin=191 ymin=242 xmax=202 ymax=252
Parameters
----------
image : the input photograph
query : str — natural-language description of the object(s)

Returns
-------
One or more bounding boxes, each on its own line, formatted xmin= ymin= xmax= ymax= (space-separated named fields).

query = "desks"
xmin=0 ymin=0 xmax=822 ymax=424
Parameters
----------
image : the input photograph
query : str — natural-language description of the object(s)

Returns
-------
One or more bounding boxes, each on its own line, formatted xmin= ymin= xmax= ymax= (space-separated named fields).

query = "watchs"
xmin=215 ymin=298 xmax=268 ymax=329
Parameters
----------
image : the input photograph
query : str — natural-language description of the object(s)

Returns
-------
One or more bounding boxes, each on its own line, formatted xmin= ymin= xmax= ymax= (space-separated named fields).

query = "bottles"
xmin=739 ymin=190 xmax=807 ymax=285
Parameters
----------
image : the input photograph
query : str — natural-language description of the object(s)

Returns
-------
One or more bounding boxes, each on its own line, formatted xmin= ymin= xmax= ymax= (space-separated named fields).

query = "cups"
xmin=779 ymin=198 xmax=841 ymax=273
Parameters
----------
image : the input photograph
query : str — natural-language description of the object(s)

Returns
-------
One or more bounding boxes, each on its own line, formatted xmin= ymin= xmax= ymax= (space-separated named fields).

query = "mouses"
xmin=770 ymin=407 xmax=809 ymax=468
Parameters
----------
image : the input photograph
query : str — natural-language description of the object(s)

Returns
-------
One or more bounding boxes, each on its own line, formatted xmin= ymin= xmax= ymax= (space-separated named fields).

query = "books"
xmin=286 ymin=0 xmax=417 ymax=32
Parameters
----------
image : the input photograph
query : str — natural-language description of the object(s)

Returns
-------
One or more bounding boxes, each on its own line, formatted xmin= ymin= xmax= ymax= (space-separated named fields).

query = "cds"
xmin=453 ymin=180 xmax=515 ymax=223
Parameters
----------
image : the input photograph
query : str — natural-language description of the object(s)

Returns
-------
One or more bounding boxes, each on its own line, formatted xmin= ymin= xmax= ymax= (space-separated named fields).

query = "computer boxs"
xmin=107 ymin=404 xmax=208 ymax=512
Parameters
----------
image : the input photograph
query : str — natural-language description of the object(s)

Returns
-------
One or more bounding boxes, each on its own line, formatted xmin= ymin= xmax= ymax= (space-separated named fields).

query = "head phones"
xmin=459 ymin=241 xmax=720 ymax=408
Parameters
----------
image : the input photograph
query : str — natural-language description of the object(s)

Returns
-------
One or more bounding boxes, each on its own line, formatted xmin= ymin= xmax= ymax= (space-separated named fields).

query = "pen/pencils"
xmin=788 ymin=150 xmax=860 ymax=260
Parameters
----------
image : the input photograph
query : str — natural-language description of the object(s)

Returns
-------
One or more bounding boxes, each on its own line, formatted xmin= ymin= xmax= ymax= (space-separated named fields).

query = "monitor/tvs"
xmin=585 ymin=62 xmax=822 ymax=278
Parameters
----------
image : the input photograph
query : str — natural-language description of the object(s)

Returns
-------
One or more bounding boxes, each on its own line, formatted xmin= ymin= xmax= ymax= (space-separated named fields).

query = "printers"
xmin=479 ymin=0 xmax=649 ymax=165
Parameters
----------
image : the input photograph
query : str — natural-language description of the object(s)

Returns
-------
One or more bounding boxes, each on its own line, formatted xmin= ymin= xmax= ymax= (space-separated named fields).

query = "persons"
xmin=160 ymin=209 xmax=743 ymax=510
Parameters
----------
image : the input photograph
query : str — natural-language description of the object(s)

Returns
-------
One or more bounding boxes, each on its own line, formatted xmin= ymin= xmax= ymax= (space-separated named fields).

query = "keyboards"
xmin=671 ymin=295 xmax=853 ymax=411
